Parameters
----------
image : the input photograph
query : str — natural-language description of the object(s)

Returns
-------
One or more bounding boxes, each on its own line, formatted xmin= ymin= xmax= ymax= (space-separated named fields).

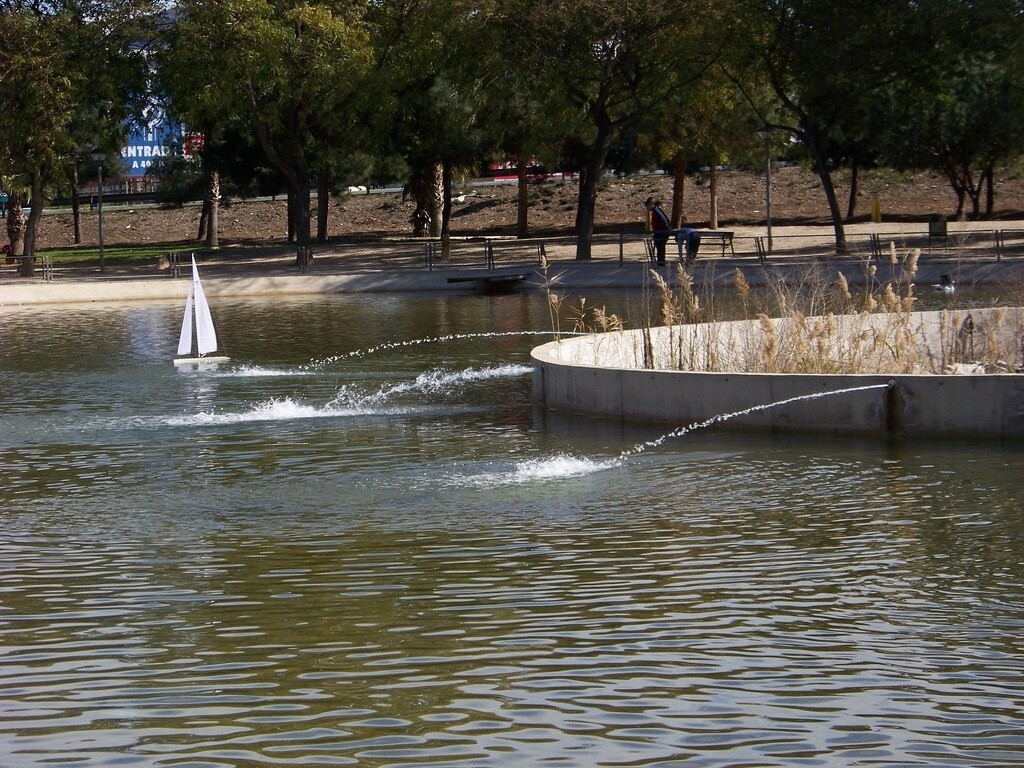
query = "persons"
xmin=645 ymin=200 xmax=673 ymax=266
xmin=675 ymin=227 xmax=700 ymax=267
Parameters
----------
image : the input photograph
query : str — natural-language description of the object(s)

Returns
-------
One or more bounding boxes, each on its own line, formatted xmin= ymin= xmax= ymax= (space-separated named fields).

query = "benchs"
xmin=699 ymin=231 xmax=735 ymax=257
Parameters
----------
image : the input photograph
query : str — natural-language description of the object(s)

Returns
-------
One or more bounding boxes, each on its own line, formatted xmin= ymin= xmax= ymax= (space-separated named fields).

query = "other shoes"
xmin=658 ymin=263 xmax=664 ymax=266
